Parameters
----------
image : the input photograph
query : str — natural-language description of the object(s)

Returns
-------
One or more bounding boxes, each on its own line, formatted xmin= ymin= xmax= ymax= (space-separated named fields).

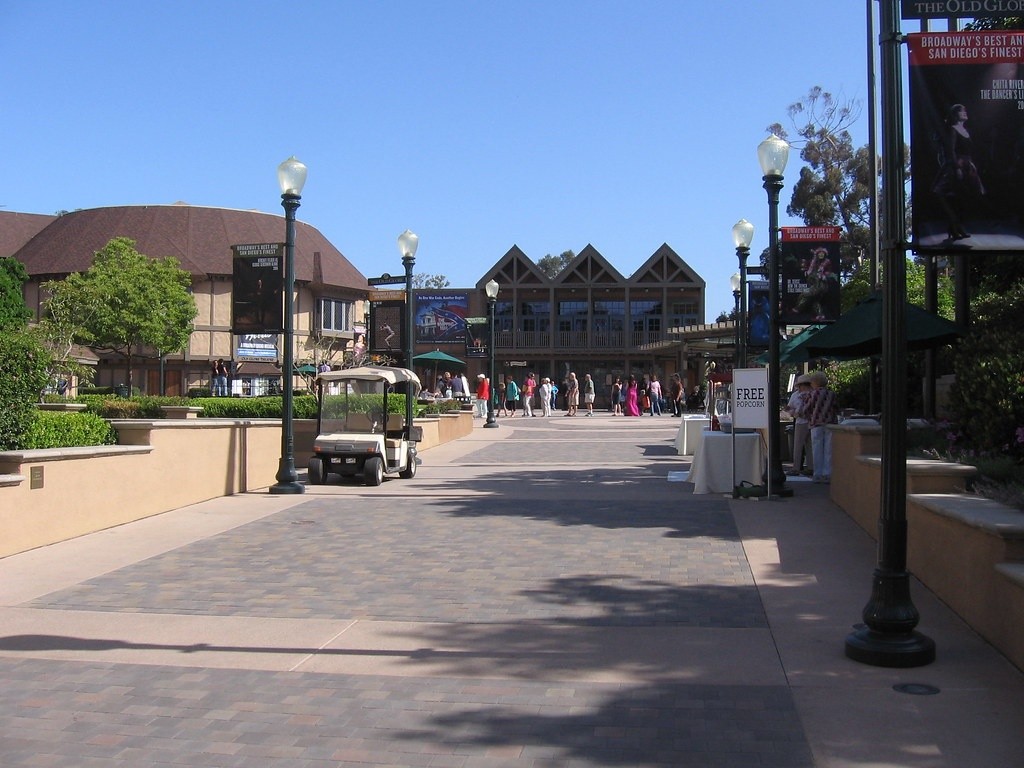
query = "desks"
xmin=673 ymin=414 xmax=796 ymax=495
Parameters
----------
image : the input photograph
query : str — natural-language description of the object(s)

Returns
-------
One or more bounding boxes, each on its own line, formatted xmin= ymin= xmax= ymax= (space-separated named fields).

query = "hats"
xmin=795 ymin=374 xmax=811 ymax=388
xmin=479 ymin=374 xmax=485 ymax=378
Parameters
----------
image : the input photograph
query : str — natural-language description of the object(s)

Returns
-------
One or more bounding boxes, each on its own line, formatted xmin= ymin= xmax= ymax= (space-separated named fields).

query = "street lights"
xmin=397 ymin=229 xmax=425 ymax=464
xmin=269 ymin=154 xmax=305 ymax=494
xmin=729 ymin=272 xmax=743 ymax=369
xmin=732 ymin=217 xmax=754 ymax=368
xmin=755 ymin=131 xmax=796 ymax=497
xmin=482 ymin=278 xmax=501 ymax=428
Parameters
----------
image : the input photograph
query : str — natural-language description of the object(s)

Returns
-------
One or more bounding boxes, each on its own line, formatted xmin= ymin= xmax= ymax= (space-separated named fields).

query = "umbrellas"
xmin=787 ymin=284 xmax=981 ymax=365
xmin=409 ymin=346 xmax=467 ymax=392
xmin=292 ymin=362 xmax=321 ymax=391
xmin=751 ymin=317 xmax=836 ymax=372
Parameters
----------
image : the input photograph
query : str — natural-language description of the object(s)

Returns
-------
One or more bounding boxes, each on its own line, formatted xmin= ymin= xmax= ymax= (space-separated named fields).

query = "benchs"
xmin=346 ymin=414 xmax=406 ymax=447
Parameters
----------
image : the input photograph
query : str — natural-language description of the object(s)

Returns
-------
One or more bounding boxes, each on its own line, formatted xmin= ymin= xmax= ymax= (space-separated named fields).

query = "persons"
xmin=943 ymin=103 xmax=985 ymax=239
xmin=254 ymin=279 xmax=266 ymax=326
xmin=475 ymin=373 xmax=495 ymax=418
xmin=495 ymin=375 xmax=522 ymax=417
xmin=671 ymin=373 xmax=682 ymax=417
xmin=610 ymin=375 xmax=623 ymax=416
xmin=792 ymin=246 xmax=831 ymax=322
xmin=521 ymin=373 xmax=537 ymax=417
xmin=379 ymin=322 xmax=395 ymax=350
xmin=419 ymin=370 xmax=465 ymax=402
xmin=784 ymin=372 xmax=838 ymax=484
xmin=645 ymin=375 xmax=662 ymax=416
xmin=538 ymin=377 xmax=559 ymax=418
xmin=564 ymin=372 xmax=580 ymax=418
xmin=686 ymin=386 xmax=702 ymax=413
xmin=210 ymin=358 xmax=229 ymax=397
xmin=584 ymin=373 xmax=596 ymax=417
xmin=625 ymin=374 xmax=641 ymax=416
xmin=55 ymin=373 xmax=69 ymax=395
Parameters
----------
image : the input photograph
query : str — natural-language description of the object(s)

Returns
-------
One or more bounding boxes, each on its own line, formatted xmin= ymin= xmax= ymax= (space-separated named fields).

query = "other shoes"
xmin=548 ymin=414 xmax=551 ymax=416
xmin=481 ymin=415 xmax=487 ymax=418
xmin=476 ymin=413 xmax=482 ymax=418
xmin=496 ymin=414 xmax=500 ymax=417
xmin=541 ymin=415 xmax=545 ymax=417
xmin=532 ymin=414 xmax=536 ymax=417
xmin=511 ymin=414 xmax=515 ymax=417
xmin=564 ymin=414 xmax=575 ymax=416
xmin=522 ymin=414 xmax=526 ymax=417
xmin=505 ymin=414 xmax=508 ymax=416
xmin=527 ymin=414 xmax=530 ymax=416
xmin=800 ymin=470 xmax=812 ymax=476
xmin=784 ymin=469 xmax=799 ymax=476
xmin=585 ymin=413 xmax=594 ymax=417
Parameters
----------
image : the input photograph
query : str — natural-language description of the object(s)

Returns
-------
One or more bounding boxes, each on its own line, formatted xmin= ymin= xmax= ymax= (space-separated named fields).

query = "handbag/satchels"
xmin=513 ymin=394 xmax=519 ymax=401
xmin=646 ymin=382 xmax=651 ymax=396
